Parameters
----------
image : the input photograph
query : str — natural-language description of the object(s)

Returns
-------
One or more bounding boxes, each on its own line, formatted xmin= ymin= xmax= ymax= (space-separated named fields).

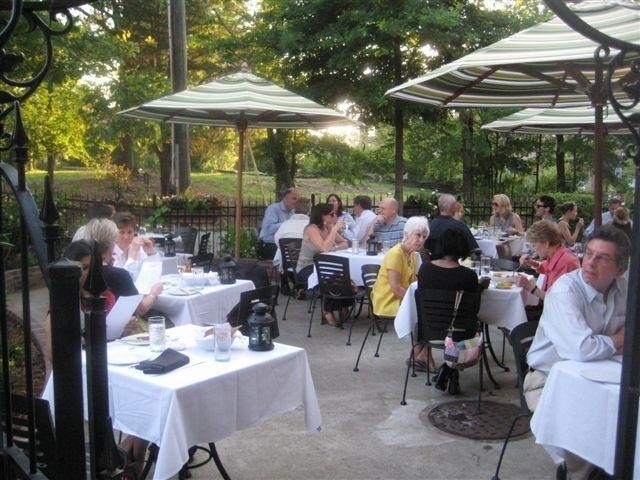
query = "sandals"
xmin=342 ymin=307 xmax=349 ymax=319
xmin=323 ymin=311 xmax=339 ymax=327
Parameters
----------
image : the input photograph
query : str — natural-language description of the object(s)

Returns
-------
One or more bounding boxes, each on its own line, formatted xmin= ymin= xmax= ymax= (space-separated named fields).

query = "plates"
xmin=196 ymin=326 xmax=242 ymax=336
xmin=342 ymin=250 xmax=352 ymax=252
xmin=475 ymin=236 xmax=484 ymax=240
xmin=195 ymin=336 xmax=235 ymax=352
xmin=106 ymin=352 xmax=151 ymax=365
xmin=495 ymin=282 xmax=511 ymax=289
xmin=167 ymin=288 xmax=203 ymax=296
xmin=492 ymin=272 xmax=519 ymax=282
xmin=122 ymin=334 xmax=175 ymax=346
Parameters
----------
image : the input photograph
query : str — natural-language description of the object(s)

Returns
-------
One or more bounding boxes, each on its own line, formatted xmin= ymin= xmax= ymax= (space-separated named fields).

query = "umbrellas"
xmin=385 ymin=0 xmax=640 ymax=236
xmin=116 ymin=61 xmax=364 ymax=260
xmin=480 ymin=107 xmax=640 ymax=134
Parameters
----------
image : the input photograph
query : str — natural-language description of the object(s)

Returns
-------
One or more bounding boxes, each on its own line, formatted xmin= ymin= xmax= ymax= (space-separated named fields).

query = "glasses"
xmin=491 ymin=203 xmax=498 ymax=207
xmin=536 ymin=205 xmax=544 ymax=209
xmin=328 ymin=212 xmax=334 ymax=217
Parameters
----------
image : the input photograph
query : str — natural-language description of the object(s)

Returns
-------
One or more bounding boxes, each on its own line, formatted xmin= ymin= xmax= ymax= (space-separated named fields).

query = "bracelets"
xmin=149 ymin=294 xmax=156 ymax=303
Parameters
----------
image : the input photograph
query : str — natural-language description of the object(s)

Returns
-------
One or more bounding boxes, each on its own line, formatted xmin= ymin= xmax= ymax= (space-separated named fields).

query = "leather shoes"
xmin=281 ymin=284 xmax=291 ymax=295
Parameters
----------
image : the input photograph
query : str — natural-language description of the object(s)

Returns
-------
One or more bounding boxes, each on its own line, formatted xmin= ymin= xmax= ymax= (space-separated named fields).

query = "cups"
xmin=381 ymin=237 xmax=391 ymax=257
xmin=481 ymin=257 xmax=490 ymax=277
xmin=138 ymin=229 xmax=146 ymax=246
xmin=352 ymin=239 xmax=361 ymax=255
xmin=214 ymin=324 xmax=231 ymax=361
xmin=471 ymin=261 xmax=480 ymax=278
xmin=148 ymin=316 xmax=166 ymax=352
xmin=335 ymin=221 xmax=346 ymax=231
xmin=175 ymin=253 xmax=192 ymax=275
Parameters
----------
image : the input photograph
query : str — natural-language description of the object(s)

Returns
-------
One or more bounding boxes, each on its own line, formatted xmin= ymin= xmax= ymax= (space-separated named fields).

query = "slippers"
xmin=414 ymin=358 xmax=439 ymax=374
xmin=405 ymin=358 xmax=413 ymax=367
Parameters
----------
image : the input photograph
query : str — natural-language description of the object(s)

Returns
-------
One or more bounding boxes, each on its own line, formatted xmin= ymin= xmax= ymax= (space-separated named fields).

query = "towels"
xmin=135 ymin=348 xmax=190 ymax=374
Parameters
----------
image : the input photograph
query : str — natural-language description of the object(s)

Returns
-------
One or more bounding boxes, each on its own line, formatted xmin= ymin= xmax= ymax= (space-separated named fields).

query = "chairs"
xmin=307 ymin=254 xmax=370 ymax=346
xmin=281 ymin=242 xmax=316 ymax=322
xmin=491 ymin=322 xmax=544 ymax=480
xmin=354 ymin=264 xmax=419 ymax=379
xmin=236 ymin=285 xmax=279 ymax=345
xmin=1 ymin=391 xmax=68 ymax=480
xmin=278 ymin=236 xmax=301 ymax=297
xmin=399 ymin=286 xmax=487 ymax=416
xmin=173 ymin=225 xmax=198 ymax=258
xmin=187 ymin=234 xmax=213 ymax=274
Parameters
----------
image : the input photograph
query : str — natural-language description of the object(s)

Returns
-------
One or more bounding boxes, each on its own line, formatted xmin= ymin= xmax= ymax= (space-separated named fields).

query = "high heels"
xmin=435 ymin=363 xmax=449 ymax=391
xmin=449 ymin=369 xmax=460 ymax=395
xmin=295 ymin=289 xmax=305 ymax=300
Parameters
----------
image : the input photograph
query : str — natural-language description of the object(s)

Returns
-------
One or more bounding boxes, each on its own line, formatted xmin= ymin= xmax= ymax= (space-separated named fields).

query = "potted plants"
xmin=216 ymin=222 xmax=259 ymax=279
xmin=162 ymin=193 xmax=224 ymax=224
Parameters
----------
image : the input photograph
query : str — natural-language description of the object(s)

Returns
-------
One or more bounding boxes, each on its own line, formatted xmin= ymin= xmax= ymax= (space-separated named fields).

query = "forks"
xmin=512 ymin=262 xmax=525 ymax=277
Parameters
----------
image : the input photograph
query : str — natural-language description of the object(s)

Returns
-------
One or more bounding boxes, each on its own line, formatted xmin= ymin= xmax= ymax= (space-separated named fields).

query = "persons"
xmin=607 ymin=207 xmax=633 ymax=234
xmin=452 ymin=202 xmax=464 ymax=221
xmin=556 ymin=201 xmax=585 ymax=247
xmin=409 ymin=225 xmax=491 ymax=395
xmin=522 ymin=223 xmax=632 ymax=480
xmin=629 ymin=211 xmax=634 ymax=221
xmin=370 ymin=216 xmax=438 ymax=372
xmin=424 ymin=193 xmax=480 ymax=260
xmin=326 ymin=193 xmax=356 ymax=223
xmin=272 ymin=197 xmax=312 ymax=295
xmin=348 ymin=195 xmax=378 ymax=248
xmin=295 ymin=202 xmax=348 ymax=327
xmin=112 ymin=211 xmax=161 ymax=281
xmin=583 ymin=200 xmax=633 ymax=237
xmin=257 ymin=187 xmax=299 ymax=259
xmin=360 ymin=197 xmax=409 ymax=248
xmin=509 ymin=220 xmax=581 ymax=389
xmin=535 ymin=196 xmax=556 ymax=221
xmin=46 ymin=239 xmax=137 ymax=364
xmin=105 ymin=202 xmax=116 ymax=218
xmin=490 ymin=193 xmax=522 ymax=232
xmin=87 ymin=218 xmax=171 ymax=323
xmin=71 ymin=205 xmax=112 ymax=242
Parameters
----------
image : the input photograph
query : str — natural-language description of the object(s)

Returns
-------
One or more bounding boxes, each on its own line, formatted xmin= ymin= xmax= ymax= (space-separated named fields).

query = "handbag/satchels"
xmin=444 ymin=291 xmax=483 ymax=371
